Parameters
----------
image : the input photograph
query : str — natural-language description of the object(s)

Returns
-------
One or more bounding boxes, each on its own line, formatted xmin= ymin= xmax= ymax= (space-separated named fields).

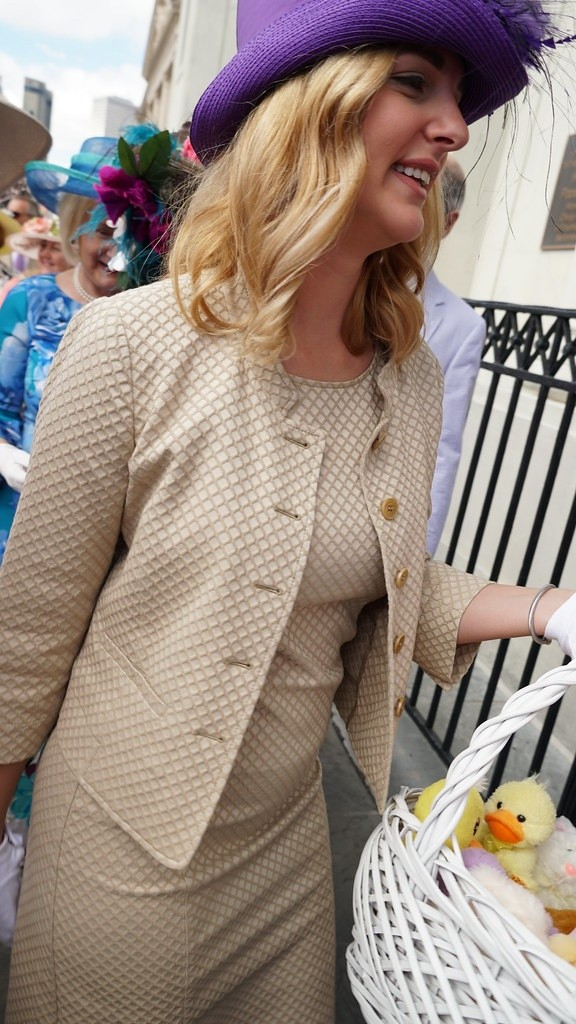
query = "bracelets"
xmin=528 ymin=582 xmax=554 ymax=645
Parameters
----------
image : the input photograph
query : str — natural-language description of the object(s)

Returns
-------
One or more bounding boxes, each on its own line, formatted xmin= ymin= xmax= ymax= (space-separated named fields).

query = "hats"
xmin=4 ymin=216 xmax=60 ymax=260
xmin=24 ymin=136 xmax=132 ymax=215
xmin=186 ymin=0 xmax=576 ymax=163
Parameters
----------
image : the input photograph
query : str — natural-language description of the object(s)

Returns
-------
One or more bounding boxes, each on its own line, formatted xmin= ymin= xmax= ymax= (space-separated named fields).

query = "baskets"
xmin=347 ymin=662 xmax=575 ymax=1023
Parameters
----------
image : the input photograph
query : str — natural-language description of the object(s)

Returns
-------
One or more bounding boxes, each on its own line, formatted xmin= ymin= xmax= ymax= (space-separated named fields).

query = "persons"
xmin=0 ymin=137 xmax=136 ymax=564
xmin=406 ymin=158 xmax=487 ymax=563
xmin=0 ymin=217 xmax=74 ymax=309
xmin=0 ymin=0 xmax=576 ymax=1024
xmin=0 ymin=211 xmax=20 ymax=257
xmin=7 ymin=196 xmax=39 ymax=224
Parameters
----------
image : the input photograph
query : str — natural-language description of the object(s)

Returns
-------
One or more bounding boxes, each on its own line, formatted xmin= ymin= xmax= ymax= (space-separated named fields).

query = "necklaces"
xmin=74 ymin=261 xmax=95 ymax=303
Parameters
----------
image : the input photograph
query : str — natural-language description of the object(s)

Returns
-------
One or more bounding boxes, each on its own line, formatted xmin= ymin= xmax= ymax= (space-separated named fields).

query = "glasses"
xmin=13 ymin=212 xmax=21 ymax=219
